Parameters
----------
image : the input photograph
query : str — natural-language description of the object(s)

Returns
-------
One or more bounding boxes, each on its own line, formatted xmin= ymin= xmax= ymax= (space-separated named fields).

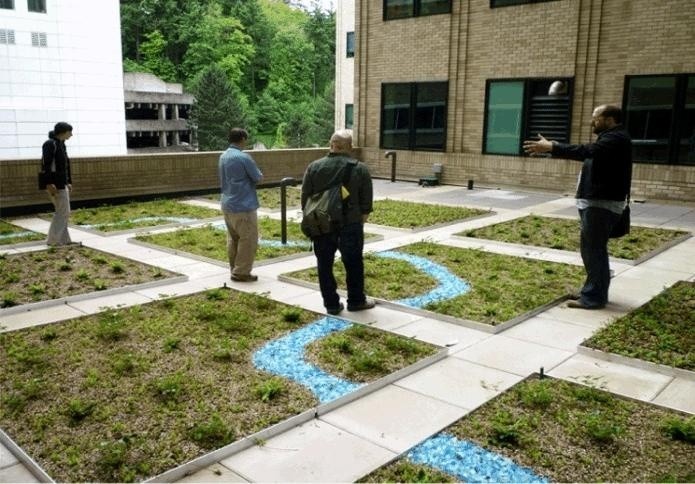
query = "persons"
xmin=217 ymin=127 xmax=264 ymax=282
xmin=522 ymin=105 xmax=633 ymax=310
xmin=40 ymin=122 xmax=75 ymax=247
xmin=300 ymin=128 xmax=377 ymax=315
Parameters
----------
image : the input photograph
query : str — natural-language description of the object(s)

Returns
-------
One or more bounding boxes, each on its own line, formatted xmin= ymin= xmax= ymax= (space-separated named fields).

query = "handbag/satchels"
xmin=38 ymin=172 xmax=65 ymax=190
xmin=609 ymin=206 xmax=629 ymax=238
xmin=301 ymin=182 xmax=346 ymax=236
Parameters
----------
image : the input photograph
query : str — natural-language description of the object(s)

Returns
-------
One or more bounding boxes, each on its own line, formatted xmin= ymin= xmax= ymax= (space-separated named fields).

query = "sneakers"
xmin=567 ymin=293 xmax=600 ymax=309
xmin=348 ymin=299 xmax=376 ymax=311
xmin=232 ymin=273 xmax=257 ymax=282
xmin=328 ymin=303 xmax=343 ymax=315
